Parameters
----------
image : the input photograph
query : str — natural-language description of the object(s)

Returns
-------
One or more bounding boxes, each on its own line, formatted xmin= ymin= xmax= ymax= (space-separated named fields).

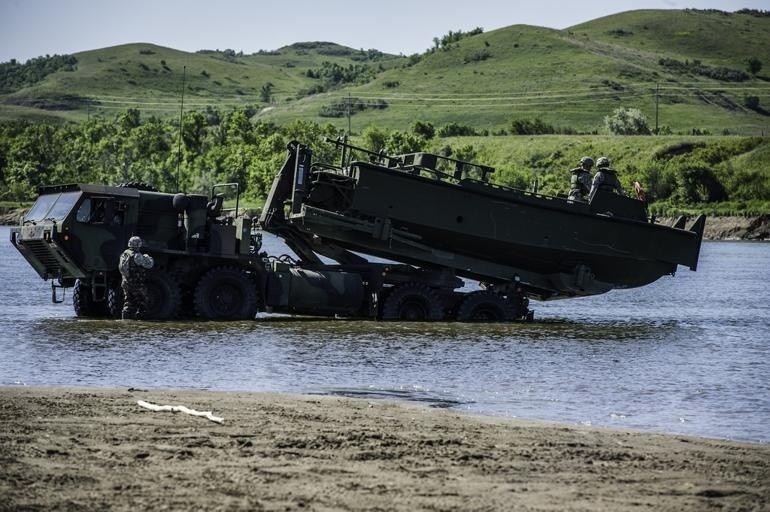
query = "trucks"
xmin=9 ymin=134 xmax=706 ymax=321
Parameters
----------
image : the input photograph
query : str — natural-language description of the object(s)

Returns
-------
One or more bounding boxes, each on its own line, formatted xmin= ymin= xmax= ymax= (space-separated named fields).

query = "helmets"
xmin=596 ymin=156 xmax=609 ymax=167
xmin=127 ymin=236 xmax=143 ymax=247
xmin=581 ymin=156 xmax=594 ymax=165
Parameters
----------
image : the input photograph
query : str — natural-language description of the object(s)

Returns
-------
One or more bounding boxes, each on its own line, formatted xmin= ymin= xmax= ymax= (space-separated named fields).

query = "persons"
xmin=587 ymin=156 xmax=627 ymax=206
xmin=118 ymin=236 xmax=155 ymax=321
xmin=565 ymin=156 xmax=595 ymax=205
